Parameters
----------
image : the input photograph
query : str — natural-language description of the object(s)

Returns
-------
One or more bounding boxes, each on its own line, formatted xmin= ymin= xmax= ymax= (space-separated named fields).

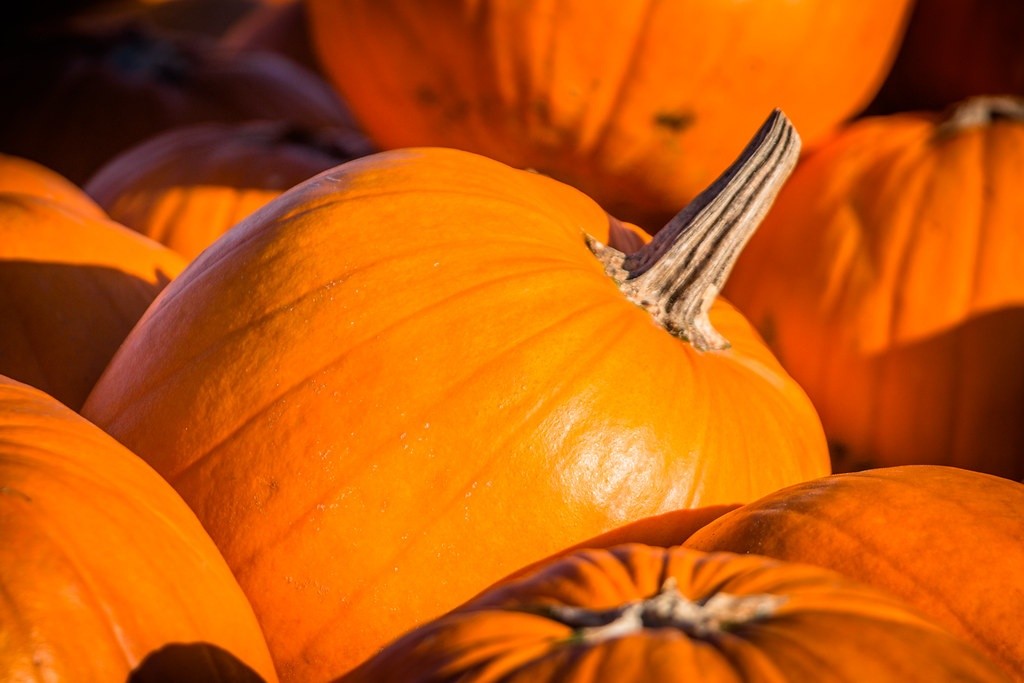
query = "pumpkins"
xmin=0 ymin=0 xmax=1024 ymax=683
